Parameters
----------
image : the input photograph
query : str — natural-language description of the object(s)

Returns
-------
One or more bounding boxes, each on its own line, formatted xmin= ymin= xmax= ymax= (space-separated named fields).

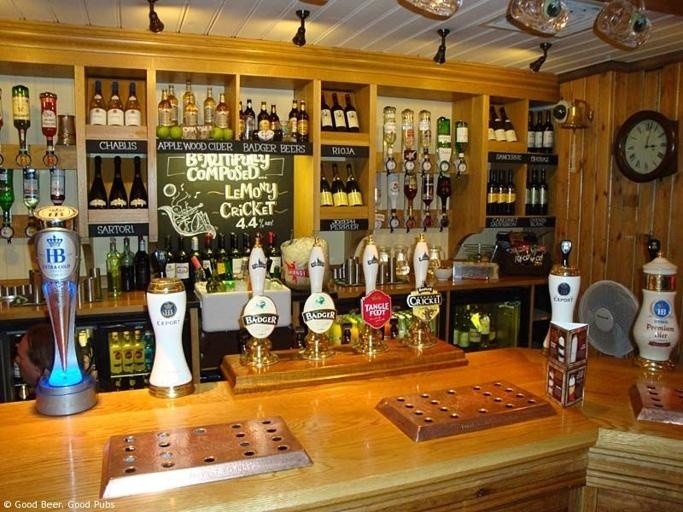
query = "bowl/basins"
xmin=433 ymin=267 xmax=452 ymax=283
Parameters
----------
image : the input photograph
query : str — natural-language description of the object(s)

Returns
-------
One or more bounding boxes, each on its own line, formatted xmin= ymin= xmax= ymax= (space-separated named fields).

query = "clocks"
xmin=611 ymin=109 xmax=678 ymax=184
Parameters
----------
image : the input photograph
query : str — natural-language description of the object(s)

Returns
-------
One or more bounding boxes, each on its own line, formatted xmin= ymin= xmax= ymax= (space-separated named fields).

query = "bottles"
xmin=375 ymin=104 xmax=467 ymax=232
xmin=10 ymin=332 xmax=33 ymax=400
xmin=0 ymin=267 xmax=102 ymax=310
xmin=378 ymin=245 xmax=442 ymax=287
xmin=155 ymin=228 xmax=284 ymax=295
xmin=452 ymin=301 xmax=496 ymax=350
xmin=487 ymin=106 xmax=553 ymax=218
xmin=77 ymin=329 xmax=94 ymax=374
xmin=0 ymin=82 xmax=69 ymax=234
xmin=89 ymin=79 xmax=141 ymax=127
xmin=158 ymin=75 xmax=310 ymax=145
xmin=108 ymin=329 xmax=153 ymax=373
xmin=88 ymin=154 xmax=153 ymax=295
xmin=319 ymin=88 xmax=365 ymax=209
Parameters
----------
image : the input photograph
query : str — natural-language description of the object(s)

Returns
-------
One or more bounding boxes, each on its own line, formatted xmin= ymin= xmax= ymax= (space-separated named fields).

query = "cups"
xmin=333 ymin=257 xmax=360 ymax=286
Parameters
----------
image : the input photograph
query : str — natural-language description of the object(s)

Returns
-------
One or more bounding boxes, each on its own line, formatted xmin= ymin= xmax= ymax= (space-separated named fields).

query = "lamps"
xmin=145 ymin=0 xmax=164 ymax=34
xmin=433 ymin=27 xmax=450 ymax=65
xmin=526 ymin=42 xmax=551 ymax=73
xmin=559 ymin=98 xmax=595 ymax=133
xmin=291 ymin=10 xmax=310 ymax=47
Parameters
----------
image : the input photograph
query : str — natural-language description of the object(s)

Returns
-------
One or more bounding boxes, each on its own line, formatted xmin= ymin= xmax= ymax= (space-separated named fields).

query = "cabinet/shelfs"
xmin=72 ymin=65 xmax=161 ymax=246
xmin=153 ymin=69 xmax=313 ymax=157
xmin=446 ymin=95 xmax=557 ymax=257
xmin=292 ymin=80 xmax=376 ymax=240
xmin=447 ymin=279 xmax=550 ymax=353
xmin=332 ymin=289 xmax=448 ymax=346
xmin=0 ymin=307 xmax=193 ymax=404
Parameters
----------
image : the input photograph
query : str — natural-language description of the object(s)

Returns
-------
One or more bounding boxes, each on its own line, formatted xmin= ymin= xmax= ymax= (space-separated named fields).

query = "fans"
xmin=577 ymin=278 xmax=642 ymax=360
